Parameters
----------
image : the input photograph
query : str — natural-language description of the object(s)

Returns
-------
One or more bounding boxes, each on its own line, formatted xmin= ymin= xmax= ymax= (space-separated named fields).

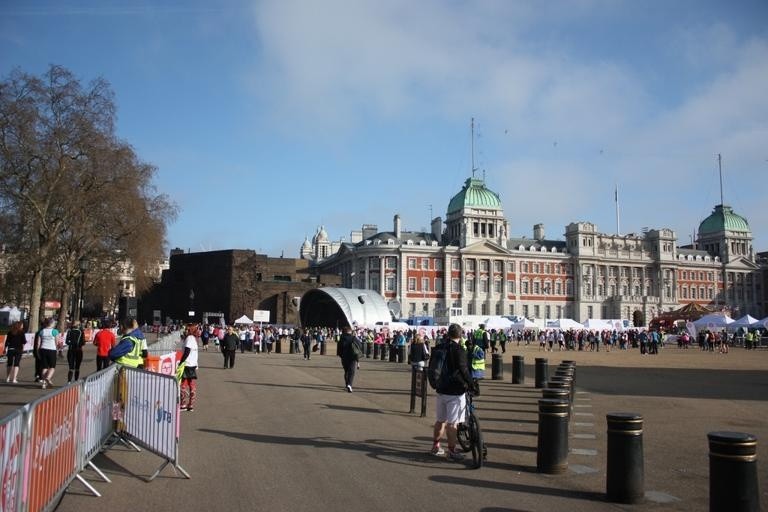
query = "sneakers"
xmin=445 ymin=448 xmax=465 ymax=463
xmin=180 ymin=407 xmax=193 ymax=412
xmin=346 ymin=384 xmax=354 ymax=394
xmin=430 ymin=446 xmax=444 ymax=456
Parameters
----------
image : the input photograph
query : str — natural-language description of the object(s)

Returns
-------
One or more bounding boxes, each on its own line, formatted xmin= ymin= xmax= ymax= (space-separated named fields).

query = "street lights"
xmin=117 ymin=279 xmax=125 ymax=336
xmin=75 ymin=252 xmax=88 ymax=329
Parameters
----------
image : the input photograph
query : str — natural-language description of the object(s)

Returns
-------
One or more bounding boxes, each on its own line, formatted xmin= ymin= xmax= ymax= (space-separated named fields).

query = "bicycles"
xmin=454 ymin=379 xmax=484 ymax=471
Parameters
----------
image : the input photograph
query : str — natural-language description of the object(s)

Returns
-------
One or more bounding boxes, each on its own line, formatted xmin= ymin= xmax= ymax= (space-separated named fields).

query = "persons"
xmin=465 ymin=340 xmax=486 ymax=383
xmin=337 ymin=327 xmax=361 ymax=392
xmin=2 ymin=315 xmax=147 ymax=389
xmin=429 ymin=324 xmax=481 ymax=462
xmin=151 ymin=323 xmax=342 ymax=368
xmin=177 ymin=324 xmax=199 ymax=413
xmin=408 ymin=334 xmax=429 ymax=372
xmin=352 ymin=325 xmax=767 ymax=362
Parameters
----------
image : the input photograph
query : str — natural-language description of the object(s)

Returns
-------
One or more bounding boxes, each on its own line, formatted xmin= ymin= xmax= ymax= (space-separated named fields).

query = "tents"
xmin=690 ymin=316 xmax=768 ymax=333
